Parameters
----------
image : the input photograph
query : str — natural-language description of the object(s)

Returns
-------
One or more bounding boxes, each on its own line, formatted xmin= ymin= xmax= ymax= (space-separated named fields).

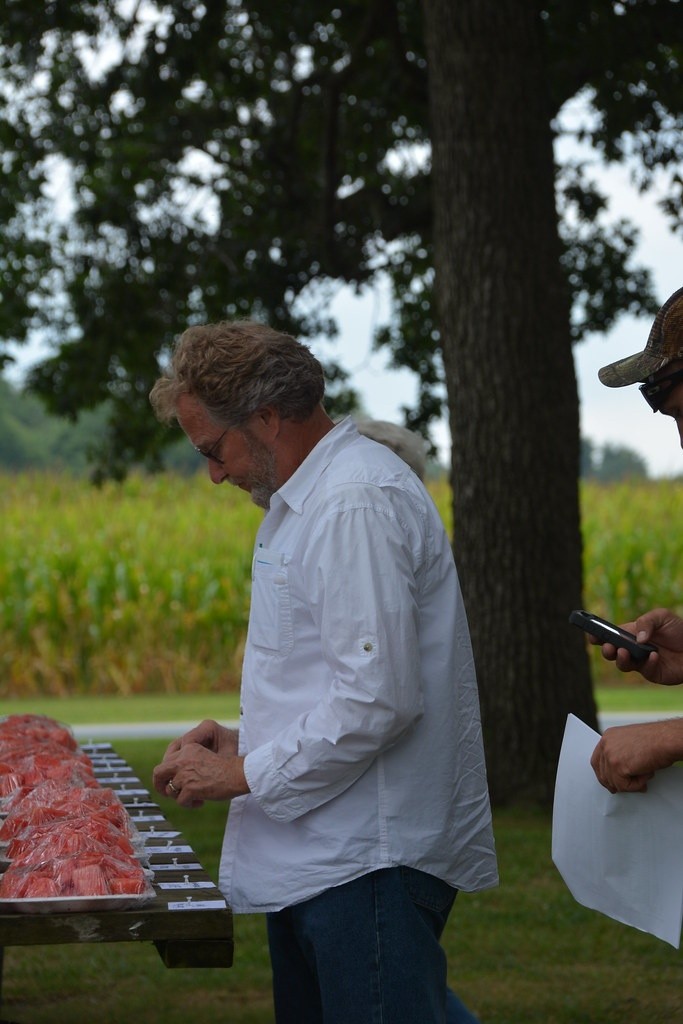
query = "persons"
xmin=150 ymin=322 xmax=503 ymax=1024
xmin=587 ymin=284 xmax=681 ymax=797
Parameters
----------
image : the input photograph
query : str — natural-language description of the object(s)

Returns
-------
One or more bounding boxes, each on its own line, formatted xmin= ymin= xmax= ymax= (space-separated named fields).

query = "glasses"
xmin=199 ymin=424 xmax=235 ymax=466
xmin=639 ymin=369 xmax=683 ymax=413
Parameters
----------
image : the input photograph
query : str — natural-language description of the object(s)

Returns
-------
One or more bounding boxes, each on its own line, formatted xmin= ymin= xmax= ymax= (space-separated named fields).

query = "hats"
xmin=598 ymin=288 xmax=683 ymax=387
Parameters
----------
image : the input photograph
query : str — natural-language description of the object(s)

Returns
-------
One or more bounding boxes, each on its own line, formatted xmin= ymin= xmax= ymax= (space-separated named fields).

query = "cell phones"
xmin=568 ymin=610 xmax=659 ymax=659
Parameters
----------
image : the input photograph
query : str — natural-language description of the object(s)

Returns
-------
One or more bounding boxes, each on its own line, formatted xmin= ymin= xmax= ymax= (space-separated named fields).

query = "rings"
xmin=168 ymin=780 xmax=180 ymax=795
